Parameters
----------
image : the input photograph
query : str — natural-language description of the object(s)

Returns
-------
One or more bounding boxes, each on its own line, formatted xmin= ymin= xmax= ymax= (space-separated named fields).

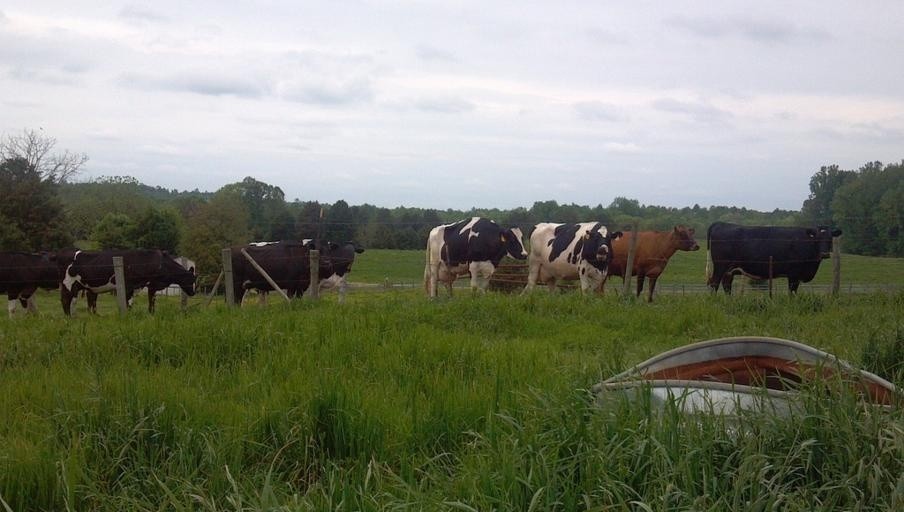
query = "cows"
xmin=0 ymin=246 xmax=202 ymax=320
xmin=596 ymin=224 xmax=699 ymax=304
xmin=705 ymin=221 xmax=843 ymax=297
xmin=517 ymin=220 xmax=625 ymax=298
xmin=224 ymin=236 xmax=365 ymax=307
xmin=422 ymin=216 xmax=529 ymax=300
